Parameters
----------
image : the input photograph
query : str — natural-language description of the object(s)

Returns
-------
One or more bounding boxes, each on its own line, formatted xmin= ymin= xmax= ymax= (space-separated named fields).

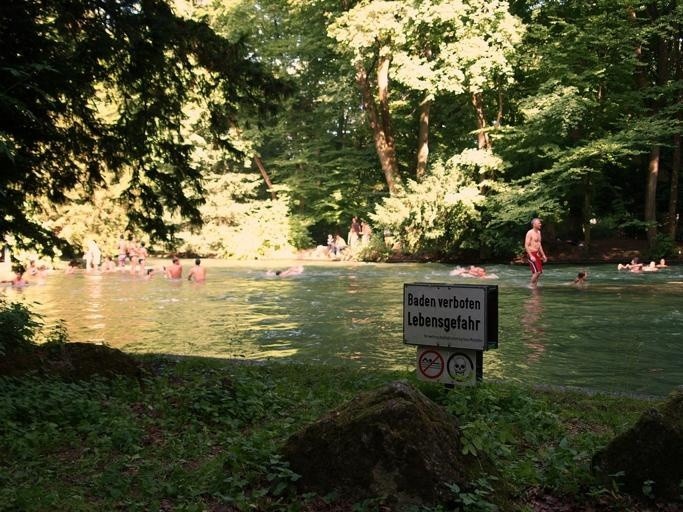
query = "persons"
xmin=617 ymin=257 xmax=667 ymax=273
xmin=574 ymin=271 xmax=587 ymax=286
xmin=0 ymin=233 xmax=207 ymax=288
xmin=326 ymin=217 xmax=370 ymax=261
xmin=276 ymin=267 xmax=295 ymax=277
xmin=524 ymin=218 xmax=548 ymax=289
xmin=450 ymin=265 xmax=486 ymax=279
